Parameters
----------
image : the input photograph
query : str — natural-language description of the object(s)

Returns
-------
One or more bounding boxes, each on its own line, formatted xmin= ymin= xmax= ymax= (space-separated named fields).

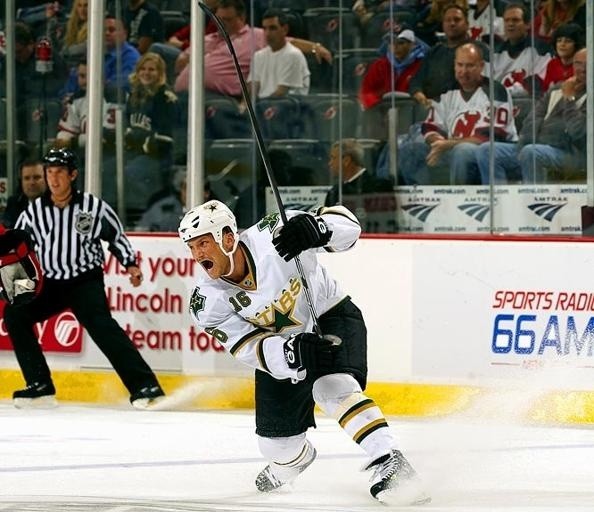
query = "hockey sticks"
xmin=198 ymin=1 xmax=323 ymax=339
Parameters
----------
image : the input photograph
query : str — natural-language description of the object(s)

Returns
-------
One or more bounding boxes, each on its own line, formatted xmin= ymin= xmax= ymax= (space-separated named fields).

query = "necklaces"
xmin=51 ymin=192 xmax=73 ymax=201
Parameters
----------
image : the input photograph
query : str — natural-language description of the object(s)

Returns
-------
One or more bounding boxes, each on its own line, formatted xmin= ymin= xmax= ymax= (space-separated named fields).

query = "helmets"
xmin=177 ymin=199 xmax=239 ymax=278
xmin=42 ymin=144 xmax=80 ymax=168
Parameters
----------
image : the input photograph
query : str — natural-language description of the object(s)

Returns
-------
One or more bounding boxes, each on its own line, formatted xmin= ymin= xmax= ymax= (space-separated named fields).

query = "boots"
xmin=255 ymin=440 xmax=319 ymax=493
xmin=361 ymin=448 xmax=433 ymax=508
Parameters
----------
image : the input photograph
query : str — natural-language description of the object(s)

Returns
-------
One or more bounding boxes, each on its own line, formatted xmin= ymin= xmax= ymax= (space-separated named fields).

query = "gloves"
xmin=281 ymin=329 xmax=347 ymax=370
xmin=272 ymin=213 xmax=334 ymax=263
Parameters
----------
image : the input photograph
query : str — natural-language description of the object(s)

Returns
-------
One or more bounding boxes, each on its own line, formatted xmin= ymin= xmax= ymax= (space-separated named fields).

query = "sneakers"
xmin=129 ymin=383 xmax=168 ymax=411
xmin=13 ymin=378 xmax=57 ymax=410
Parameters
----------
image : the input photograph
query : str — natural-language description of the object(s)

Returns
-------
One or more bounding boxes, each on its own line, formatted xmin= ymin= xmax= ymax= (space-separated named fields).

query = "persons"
xmin=2 ymin=144 xmax=167 ymax=410
xmin=323 ymin=136 xmax=399 ymax=234
xmin=1 ymin=1 xmax=334 ymax=162
xmin=134 ymin=168 xmax=219 ymax=232
xmin=1 ymin=154 xmax=50 ymax=228
xmin=176 ymin=198 xmax=431 ymax=506
xmin=350 ymin=1 xmax=586 ymax=185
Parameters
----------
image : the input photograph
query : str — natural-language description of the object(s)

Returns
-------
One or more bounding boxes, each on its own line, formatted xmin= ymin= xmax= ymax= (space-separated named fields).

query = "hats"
xmin=382 ymin=22 xmax=416 ymax=45
